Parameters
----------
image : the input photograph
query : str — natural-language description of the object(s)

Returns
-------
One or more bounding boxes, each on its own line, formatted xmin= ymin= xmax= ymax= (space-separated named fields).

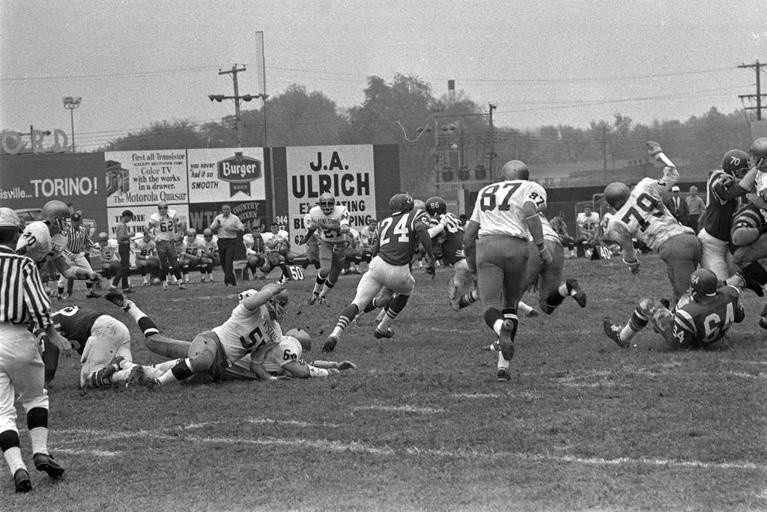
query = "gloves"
xmin=645 ymin=141 xmax=663 ymax=160
xmin=540 ymin=245 xmax=553 ymax=265
xmin=622 ymin=259 xmax=640 ymax=276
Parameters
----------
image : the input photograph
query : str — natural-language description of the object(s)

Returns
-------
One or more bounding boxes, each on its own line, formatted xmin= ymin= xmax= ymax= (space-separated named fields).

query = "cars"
xmin=12 ymin=208 xmax=98 ymax=236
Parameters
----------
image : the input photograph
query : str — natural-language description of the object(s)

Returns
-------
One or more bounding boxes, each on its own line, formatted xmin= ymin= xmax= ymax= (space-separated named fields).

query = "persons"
xmin=568 ymin=136 xmax=766 ymax=350
xmin=297 ymin=157 xmax=588 ymax=380
xmin=0 ymin=205 xmax=63 ymax=493
xmin=2 ymin=194 xmax=358 ymax=390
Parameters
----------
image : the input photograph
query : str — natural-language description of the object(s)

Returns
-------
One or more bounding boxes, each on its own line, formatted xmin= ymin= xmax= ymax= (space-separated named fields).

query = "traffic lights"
xmin=418 ymin=126 xmax=432 ymax=135
xmin=440 ymin=122 xmax=455 ymax=135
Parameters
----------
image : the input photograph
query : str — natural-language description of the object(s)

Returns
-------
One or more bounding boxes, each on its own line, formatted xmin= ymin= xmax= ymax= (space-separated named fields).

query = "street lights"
xmin=64 ymin=95 xmax=82 ymax=152
xmin=488 ymin=100 xmax=497 ymax=184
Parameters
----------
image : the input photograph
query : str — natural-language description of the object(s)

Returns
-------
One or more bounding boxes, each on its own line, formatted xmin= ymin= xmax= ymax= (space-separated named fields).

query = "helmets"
xmin=41 ymin=200 xmax=70 ymax=223
xmin=748 ymin=137 xmax=767 ymax=172
xmin=285 ymin=328 xmax=311 ymax=351
xmin=319 ymin=192 xmax=335 ymax=215
xmin=689 ymin=268 xmax=717 ymax=302
xmin=389 ymin=193 xmax=446 ymax=216
xmin=260 ymin=283 xmax=289 ymax=321
xmin=501 ymin=160 xmax=528 ymax=181
xmin=722 ymin=150 xmax=750 ymax=181
xmin=604 ymin=182 xmax=630 ymax=210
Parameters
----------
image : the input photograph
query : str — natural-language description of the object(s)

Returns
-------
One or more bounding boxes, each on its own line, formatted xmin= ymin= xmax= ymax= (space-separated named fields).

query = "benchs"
xmin=230 ymin=258 xmax=311 ymax=279
xmin=576 ymin=234 xmax=641 ymax=259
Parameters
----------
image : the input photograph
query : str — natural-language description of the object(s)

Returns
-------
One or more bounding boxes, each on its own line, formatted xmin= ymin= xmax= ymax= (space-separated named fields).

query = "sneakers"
xmin=566 ymin=278 xmax=586 ymax=307
xmin=124 ymin=288 xmax=136 ymax=293
xmin=603 ymin=318 xmax=629 ymax=349
xmin=86 ymin=291 xmax=100 ymax=298
xmin=481 ymin=320 xmax=515 ymax=382
xmin=14 ymin=468 xmax=31 ymax=492
xmin=526 ymin=310 xmax=538 ymax=317
xmin=33 ymin=453 xmax=64 ymax=477
xmin=308 ymin=292 xmax=319 ymax=305
xmin=104 ymin=293 xmax=133 ymax=312
xmin=322 ymin=317 xmax=394 ymax=351
xmin=318 ymin=296 xmax=326 ymax=304
xmin=102 ymin=356 xmax=161 ymax=392
xmin=736 ymin=267 xmax=763 ymax=297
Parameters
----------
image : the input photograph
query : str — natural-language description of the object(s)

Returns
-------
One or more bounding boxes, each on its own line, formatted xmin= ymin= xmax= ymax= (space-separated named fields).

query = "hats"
xmin=0 ymin=207 xmax=23 ymax=233
xmin=71 ymin=213 xmax=79 ymax=221
